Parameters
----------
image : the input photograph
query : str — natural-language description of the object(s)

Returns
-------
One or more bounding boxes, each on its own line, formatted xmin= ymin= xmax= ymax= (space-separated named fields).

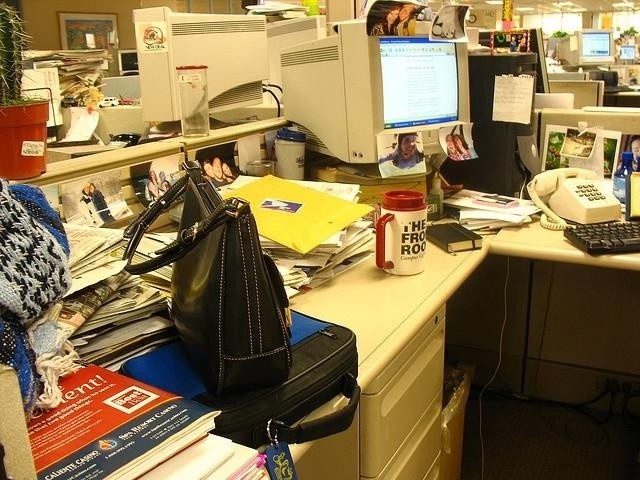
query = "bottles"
xmin=273 ymin=127 xmax=305 ymax=180
xmin=176 ymin=66 xmax=212 ymax=138
xmin=612 ymin=151 xmax=636 ymax=203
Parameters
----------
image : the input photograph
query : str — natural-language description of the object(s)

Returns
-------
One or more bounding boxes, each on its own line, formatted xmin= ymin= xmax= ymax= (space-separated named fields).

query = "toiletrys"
xmin=428 ymin=178 xmax=444 ymax=220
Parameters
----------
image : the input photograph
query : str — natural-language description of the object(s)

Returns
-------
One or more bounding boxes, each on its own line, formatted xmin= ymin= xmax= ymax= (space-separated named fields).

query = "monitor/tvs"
xmin=559 ymin=30 xmax=616 ymax=66
xmin=620 ymin=45 xmax=637 ymax=61
xmin=117 ymin=49 xmax=139 ymax=76
xmin=279 ymin=19 xmax=471 ymax=165
xmin=262 ymin=14 xmax=328 ymax=87
xmin=133 ymin=6 xmax=270 ymax=124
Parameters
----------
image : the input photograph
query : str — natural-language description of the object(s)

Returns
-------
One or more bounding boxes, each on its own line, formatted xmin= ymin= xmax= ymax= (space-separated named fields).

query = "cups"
xmin=246 ymin=160 xmax=275 ymax=176
xmin=375 ymin=190 xmax=428 ymax=275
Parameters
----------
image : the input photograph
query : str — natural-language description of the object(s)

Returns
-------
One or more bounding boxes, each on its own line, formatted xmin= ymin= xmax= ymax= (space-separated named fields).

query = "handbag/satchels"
xmin=119 ymin=299 xmax=361 ymax=446
xmin=118 ymin=166 xmax=298 ymax=397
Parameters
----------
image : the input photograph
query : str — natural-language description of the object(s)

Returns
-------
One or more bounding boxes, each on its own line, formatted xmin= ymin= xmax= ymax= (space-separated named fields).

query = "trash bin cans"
xmin=441 ymin=363 xmax=476 ymax=480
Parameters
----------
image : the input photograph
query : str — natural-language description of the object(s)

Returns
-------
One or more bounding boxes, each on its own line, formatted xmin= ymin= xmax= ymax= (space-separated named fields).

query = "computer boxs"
xmin=440 ymin=51 xmax=539 ymax=198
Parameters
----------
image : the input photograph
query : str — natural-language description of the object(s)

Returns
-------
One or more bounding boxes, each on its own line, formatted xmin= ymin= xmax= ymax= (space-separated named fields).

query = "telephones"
xmin=533 ymin=167 xmax=622 ymax=225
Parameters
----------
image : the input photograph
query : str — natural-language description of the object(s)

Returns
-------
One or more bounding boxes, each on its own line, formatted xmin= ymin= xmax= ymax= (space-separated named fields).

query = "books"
xmin=0 ymin=314 xmax=267 ymax=480
xmin=426 ymin=188 xmax=542 ymax=256
xmin=20 ymin=48 xmax=113 ymax=101
xmin=245 ymin=3 xmax=309 ymax=23
xmin=169 ymin=177 xmax=376 ymax=290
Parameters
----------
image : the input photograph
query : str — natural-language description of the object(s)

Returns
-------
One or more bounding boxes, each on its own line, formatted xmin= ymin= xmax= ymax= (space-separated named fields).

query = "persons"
xmin=202 ymin=157 xmax=236 ymax=187
xmin=159 ymin=172 xmax=171 ymax=193
xmin=90 ymin=183 xmax=116 ymax=224
xmin=79 ymin=184 xmax=105 ymax=228
xmin=393 ymin=3 xmax=417 ymax=37
xmin=446 ymin=134 xmax=464 ymax=161
xmin=616 ymin=135 xmax=640 ymax=179
xmin=369 ymin=8 xmax=400 ymax=36
xmin=453 ymin=134 xmax=472 ymax=160
xmin=392 ymin=132 xmax=424 ymax=169
xmin=147 ymin=170 xmax=161 ymax=201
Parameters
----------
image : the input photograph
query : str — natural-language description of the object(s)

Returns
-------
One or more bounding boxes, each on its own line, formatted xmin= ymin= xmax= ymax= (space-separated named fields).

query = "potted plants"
xmin=0 ymin=1 xmax=55 ymax=184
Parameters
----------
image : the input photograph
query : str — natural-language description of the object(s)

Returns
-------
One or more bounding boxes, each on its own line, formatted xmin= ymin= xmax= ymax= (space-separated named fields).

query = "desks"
xmin=126 ymin=194 xmax=498 ymax=480
xmin=543 ymin=59 xmax=640 ymax=113
xmin=487 ymin=206 xmax=640 ymax=273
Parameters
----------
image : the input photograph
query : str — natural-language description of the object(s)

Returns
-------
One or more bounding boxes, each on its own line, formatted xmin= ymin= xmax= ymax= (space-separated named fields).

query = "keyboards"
xmin=563 ymin=219 xmax=640 ymax=254
